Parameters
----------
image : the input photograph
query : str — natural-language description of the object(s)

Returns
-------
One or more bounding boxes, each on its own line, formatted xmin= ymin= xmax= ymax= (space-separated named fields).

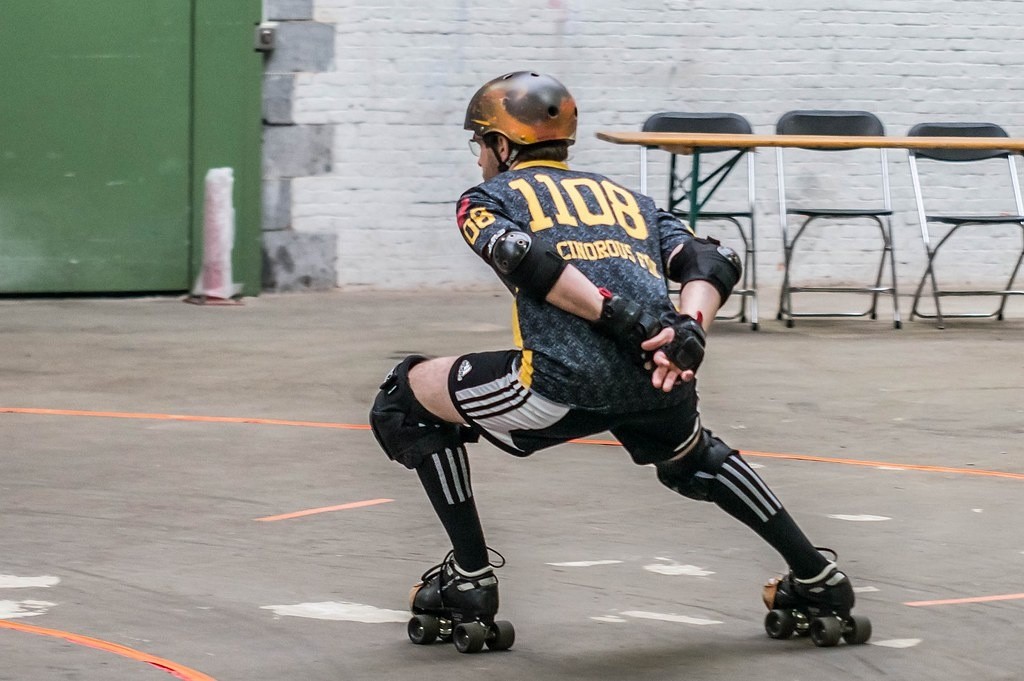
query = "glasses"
xmin=468 ymin=138 xmax=481 ymax=157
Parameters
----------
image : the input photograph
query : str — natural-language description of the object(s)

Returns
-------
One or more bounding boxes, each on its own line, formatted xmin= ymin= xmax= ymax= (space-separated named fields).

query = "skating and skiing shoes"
xmin=762 ymin=559 xmax=872 ymax=646
xmin=407 ymin=554 xmax=515 ymax=654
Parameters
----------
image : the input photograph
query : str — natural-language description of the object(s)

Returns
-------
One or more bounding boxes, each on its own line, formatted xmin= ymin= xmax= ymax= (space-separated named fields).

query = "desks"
xmin=597 ymin=131 xmax=1024 ymax=238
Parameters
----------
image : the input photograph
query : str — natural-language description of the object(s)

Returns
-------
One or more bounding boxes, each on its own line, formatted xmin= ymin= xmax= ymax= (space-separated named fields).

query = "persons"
xmin=368 ymin=70 xmax=873 ymax=655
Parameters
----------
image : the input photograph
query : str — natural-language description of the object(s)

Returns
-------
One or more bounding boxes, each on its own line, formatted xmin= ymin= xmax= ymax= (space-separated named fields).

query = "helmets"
xmin=464 ymin=71 xmax=578 ymax=145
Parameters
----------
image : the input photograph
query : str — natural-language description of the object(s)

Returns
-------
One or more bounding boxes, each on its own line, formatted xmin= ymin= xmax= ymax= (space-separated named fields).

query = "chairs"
xmin=909 ymin=120 xmax=1024 ymax=331
xmin=637 ymin=111 xmax=762 ymax=331
xmin=778 ymin=111 xmax=902 ymax=330
